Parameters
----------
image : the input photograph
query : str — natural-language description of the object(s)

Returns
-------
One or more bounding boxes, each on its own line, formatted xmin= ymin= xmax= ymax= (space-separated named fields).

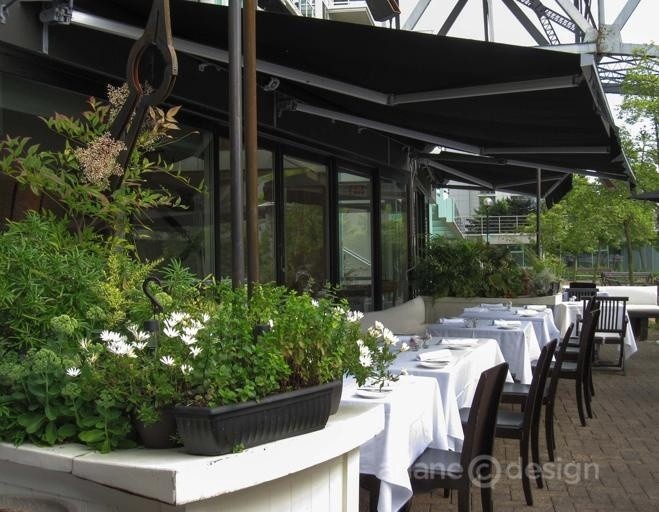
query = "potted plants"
xmin=0 ymin=84 xmax=417 ymax=458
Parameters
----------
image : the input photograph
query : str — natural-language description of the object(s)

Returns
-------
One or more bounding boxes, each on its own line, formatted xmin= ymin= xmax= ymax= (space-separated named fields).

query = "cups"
xmin=463 ymin=318 xmax=473 ymax=328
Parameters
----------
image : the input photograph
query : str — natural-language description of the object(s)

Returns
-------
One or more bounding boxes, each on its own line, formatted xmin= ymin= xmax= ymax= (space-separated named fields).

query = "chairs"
xmin=360 ymin=296 xmax=629 ymax=512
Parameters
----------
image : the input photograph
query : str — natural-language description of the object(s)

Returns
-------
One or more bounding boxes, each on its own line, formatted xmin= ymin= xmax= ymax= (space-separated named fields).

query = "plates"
xmin=517 ymin=310 xmax=538 ymax=317
xmin=419 ymin=359 xmax=450 ymax=369
xmin=356 ymin=384 xmax=393 ymax=398
xmin=495 ymin=321 xmax=521 ymax=330
xmin=447 ymin=343 xmax=473 ymax=349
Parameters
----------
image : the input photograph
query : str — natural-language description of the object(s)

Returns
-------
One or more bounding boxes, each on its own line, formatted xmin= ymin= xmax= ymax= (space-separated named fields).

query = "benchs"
xmin=598 ymin=285 xmax=659 ymax=340
